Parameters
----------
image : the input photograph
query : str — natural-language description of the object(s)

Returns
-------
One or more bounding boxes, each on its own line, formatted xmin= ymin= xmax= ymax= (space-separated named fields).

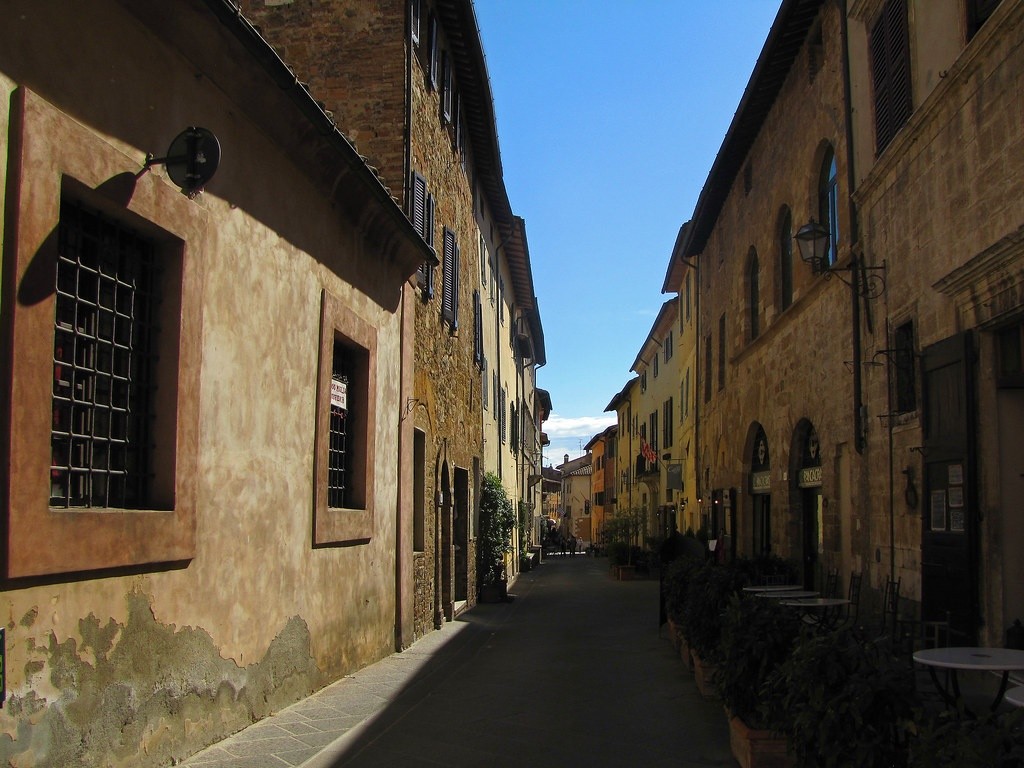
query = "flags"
xmin=558 ymin=508 xmax=567 ymax=516
xmin=640 ymin=436 xmax=656 ymax=463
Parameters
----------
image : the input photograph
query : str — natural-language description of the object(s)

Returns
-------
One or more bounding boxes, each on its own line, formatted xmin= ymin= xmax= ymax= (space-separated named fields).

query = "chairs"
xmin=761 ymin=566 xmax=950 ymax=686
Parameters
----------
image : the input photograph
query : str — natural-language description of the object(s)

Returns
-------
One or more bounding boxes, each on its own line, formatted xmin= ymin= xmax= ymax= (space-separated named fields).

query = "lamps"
xmin=791 ymin=217 xmax=887 ymax=299
xmin=680 ymin=497 xmax=688 ymax=507
xmin=670 ymin=503 xmax=678 ymax=512
xmin=860 ymin=346 xmax=914 ymax=378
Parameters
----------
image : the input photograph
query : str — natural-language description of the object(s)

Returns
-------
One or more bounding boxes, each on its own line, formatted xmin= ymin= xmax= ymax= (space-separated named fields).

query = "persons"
xmin=543 ymin=534 xmax=583 ymax=559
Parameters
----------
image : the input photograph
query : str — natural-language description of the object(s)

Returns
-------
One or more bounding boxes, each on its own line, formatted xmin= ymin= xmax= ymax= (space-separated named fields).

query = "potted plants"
xmin=721 ymin=611 xmax=812 ymax=768
xmin=663 ymin=554 xmax=689 ymax=641
xmin=608 ymin=507 xmax=648 ymax=581
xmin=678 ymin=560 xmax=709 ymax=668
xmin=519 ymin=499 xmax=530 ymax=572
xmin=469 ymin=472 xmax=512 ymax=604
xmin=688 ymin=557 xmax=741 ymax=700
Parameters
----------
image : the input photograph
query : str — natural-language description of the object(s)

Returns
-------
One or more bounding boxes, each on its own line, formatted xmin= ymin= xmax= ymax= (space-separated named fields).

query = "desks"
xmin=778 ymin=598 xmax=852 ymax=635
xmin=754 ymin=591 xmax=820 ymax=598
xmin=743 ymin=585 xmax=803 ymax=591
xmin=913 ymin=646 xmax=1024 ymax=719
